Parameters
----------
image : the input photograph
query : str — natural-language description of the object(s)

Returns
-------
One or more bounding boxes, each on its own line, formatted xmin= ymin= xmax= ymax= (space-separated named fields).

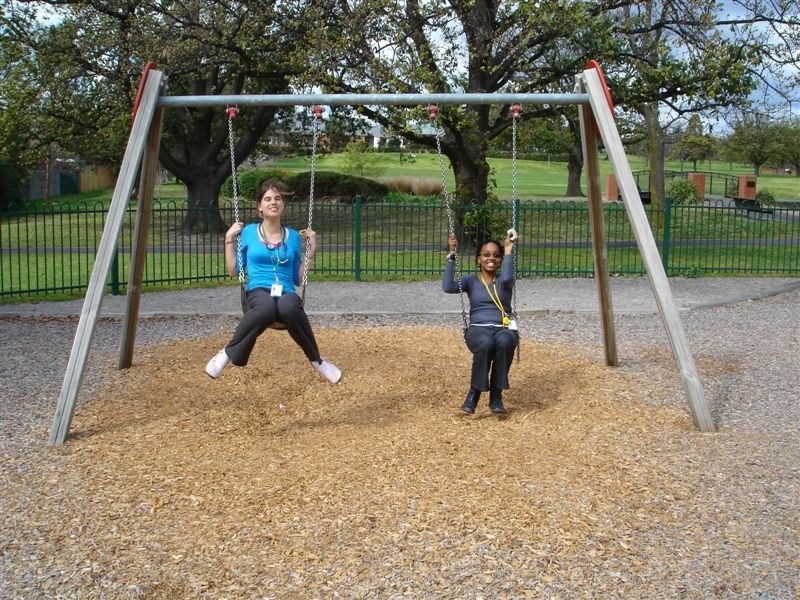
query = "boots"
xmin=461 ymin=388 xmax=480 ymax=414
xmin=489 ymin=388 xmax=505 ymax=413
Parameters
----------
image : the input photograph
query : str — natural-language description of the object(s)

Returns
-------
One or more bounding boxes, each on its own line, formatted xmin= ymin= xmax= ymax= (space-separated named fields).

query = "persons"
xmin=206 ymin=178 xmax=342 ymax=383
xmin=442 ymin=233 xmax=517 ymax=413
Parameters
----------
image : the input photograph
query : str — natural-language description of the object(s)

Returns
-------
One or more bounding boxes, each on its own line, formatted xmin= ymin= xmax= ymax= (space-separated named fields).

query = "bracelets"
xmin=225 ymin=240 xmax=236 ymax=244
xmin=446 ymin=254 xmax=456 ymax=261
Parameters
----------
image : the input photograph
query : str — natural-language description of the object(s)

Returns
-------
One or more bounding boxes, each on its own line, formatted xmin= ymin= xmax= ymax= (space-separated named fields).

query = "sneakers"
xmin=315 ymin=359 xmax=342 ymax=384
xmin=205 ymin=348 xmax=228 ymax=379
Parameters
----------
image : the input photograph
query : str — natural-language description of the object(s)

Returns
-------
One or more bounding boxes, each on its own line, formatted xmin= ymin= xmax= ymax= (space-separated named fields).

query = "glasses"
xmin=479 ymin=252 xmax=501 ymax=258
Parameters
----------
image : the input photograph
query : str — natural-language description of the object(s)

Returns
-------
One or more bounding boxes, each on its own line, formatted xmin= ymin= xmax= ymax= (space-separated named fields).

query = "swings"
xmin=225 ymin=102 xmax=324 ymax=330
xmin=425 ymin=101 xmax=525 ymax=362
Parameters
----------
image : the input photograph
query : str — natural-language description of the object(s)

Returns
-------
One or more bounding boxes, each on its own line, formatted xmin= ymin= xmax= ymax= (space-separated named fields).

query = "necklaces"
xmin=259 ymin=221 xmax=285 ymax=242
